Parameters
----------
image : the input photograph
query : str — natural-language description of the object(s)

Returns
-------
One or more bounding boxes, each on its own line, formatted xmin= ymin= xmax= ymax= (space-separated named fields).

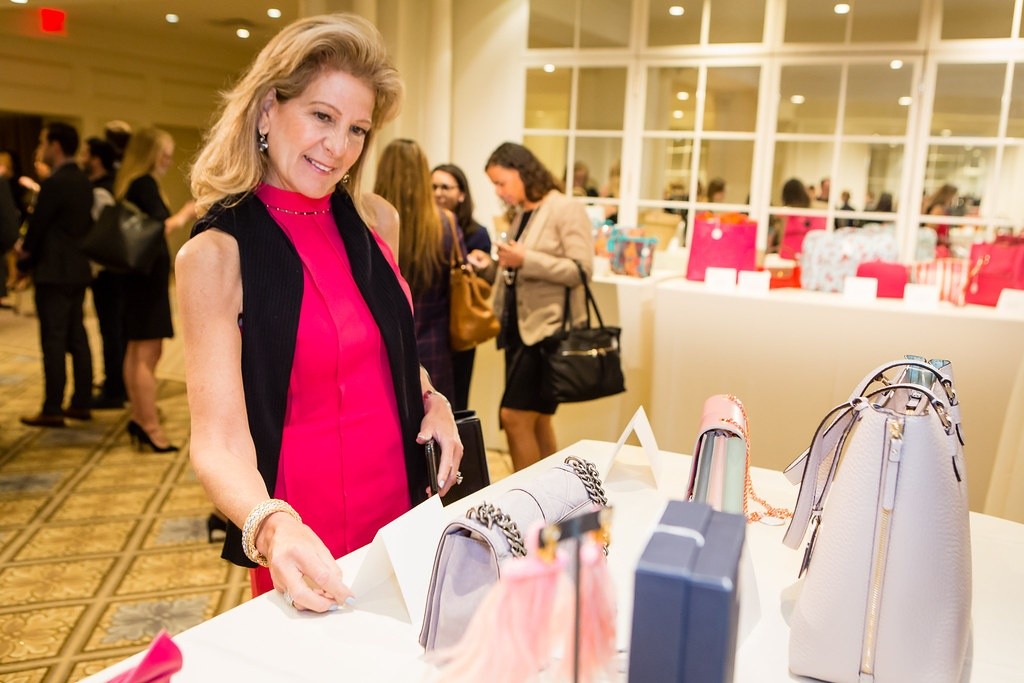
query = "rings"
xmin=284 ymin=591 xmax=293 ymax=606
xmin=457 ymin=471 xmax=463 ymax=484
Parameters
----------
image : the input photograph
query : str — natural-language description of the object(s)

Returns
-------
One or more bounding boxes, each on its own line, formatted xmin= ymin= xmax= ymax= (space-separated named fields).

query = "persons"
xmin=0 ymin=120 xmax=204 ymax=454
xmin=562 ymin=161 xmax=958 ymax=253
xmin=175 ymin=11 xmax=463 ymax=610
xmin=374 ymin=137 xmax=467 ymax=412
xmin=466 ymin=142 xmax=594 ymax=472
xmin=430 ymin=164 xmax=491 ymax=409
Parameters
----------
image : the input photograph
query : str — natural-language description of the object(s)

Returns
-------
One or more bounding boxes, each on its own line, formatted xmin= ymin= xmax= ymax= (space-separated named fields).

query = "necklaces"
xmin=264 ymin=203 xmax=331 ymax=214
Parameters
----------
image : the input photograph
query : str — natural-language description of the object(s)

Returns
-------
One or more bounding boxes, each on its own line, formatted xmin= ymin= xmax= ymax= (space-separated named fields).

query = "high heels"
xmin=127 ymin=420 xmax=180 ymax=453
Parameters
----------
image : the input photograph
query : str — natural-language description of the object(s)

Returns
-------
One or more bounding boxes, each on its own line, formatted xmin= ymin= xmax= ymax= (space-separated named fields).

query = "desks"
xmin=76 ymin=440 xmax=1024 ymax=683
xmin=595 ymin=254 xmax=1020 ymax=521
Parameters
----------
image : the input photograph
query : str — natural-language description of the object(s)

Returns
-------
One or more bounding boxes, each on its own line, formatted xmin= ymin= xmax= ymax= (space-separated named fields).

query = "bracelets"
xmin=242 ymin=498 xmax=302 ymax=567
xmin=423 ymin=390 xmax=432 ymax=409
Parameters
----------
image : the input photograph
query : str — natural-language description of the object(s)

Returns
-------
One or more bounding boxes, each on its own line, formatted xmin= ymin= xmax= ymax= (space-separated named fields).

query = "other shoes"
xmin=91 ymin=394 xmax=124 ymax=409
xmin=20 ymin=413 xmax=64 ymax=429
xmin=64 ymin=407 xmax=93 ymax=420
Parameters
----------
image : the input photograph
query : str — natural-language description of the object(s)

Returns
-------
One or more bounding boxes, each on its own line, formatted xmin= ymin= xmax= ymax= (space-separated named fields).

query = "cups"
xmin=497 ymin=231 xmax=512 ymax=243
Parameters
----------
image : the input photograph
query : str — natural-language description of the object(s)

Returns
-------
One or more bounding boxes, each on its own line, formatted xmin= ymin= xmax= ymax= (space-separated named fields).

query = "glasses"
xmin=431 ymin=184 xmax=459 ymax=194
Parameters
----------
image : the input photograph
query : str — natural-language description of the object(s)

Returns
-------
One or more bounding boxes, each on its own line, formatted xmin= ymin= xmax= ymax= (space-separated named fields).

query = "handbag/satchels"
xmin=444 ymin=208 xmax=503 ymax=352
xmin=964 ymin=234 xmax=1024 ymax=306
xmin=779 ymin=351 xmax=972 ymax=683
xmin=419 ymin=454 xmax=617 ymax=659
xmin=546 ymin=261 xmax=628 ymax=403
xmin=608 ymin=233 xmax=656 ymax=277
xmin=685 ymin=220 xmax=757 ymax=281
xmin=119 ymin=200 xmax=162 ymax=267
xmin=686 ymin=394 xmax=748 ymax=516
